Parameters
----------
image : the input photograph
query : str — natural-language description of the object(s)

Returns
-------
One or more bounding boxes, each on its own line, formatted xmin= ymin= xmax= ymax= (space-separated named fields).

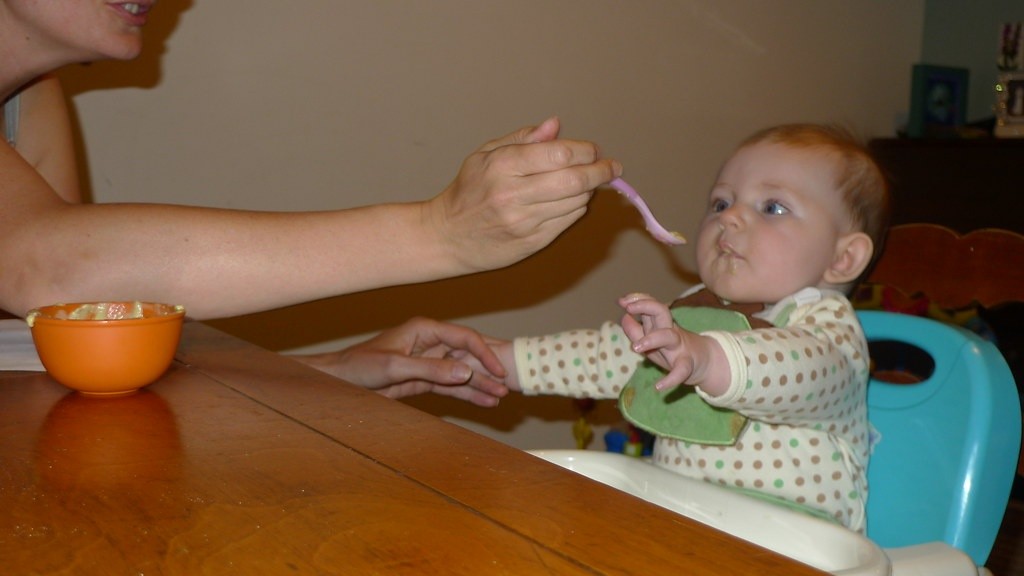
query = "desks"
xmin=0 ymin=320 xmax=833 ymax=576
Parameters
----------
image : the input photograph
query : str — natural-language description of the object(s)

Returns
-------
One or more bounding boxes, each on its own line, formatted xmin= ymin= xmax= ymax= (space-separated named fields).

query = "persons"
xmin=443 ymin=121 xmax=889 ymax=539
xmin=0 ymin=0 xmax=624 ymax=406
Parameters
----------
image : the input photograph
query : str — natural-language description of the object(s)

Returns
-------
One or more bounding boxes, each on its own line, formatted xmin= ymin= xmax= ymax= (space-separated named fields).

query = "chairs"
xmin=852 ymin=311 xmax=1022 ymax=568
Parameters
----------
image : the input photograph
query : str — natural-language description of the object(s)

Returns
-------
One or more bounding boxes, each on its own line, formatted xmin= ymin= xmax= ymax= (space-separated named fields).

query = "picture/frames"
xmin=908 ymin=65 xmax=969 ymax=137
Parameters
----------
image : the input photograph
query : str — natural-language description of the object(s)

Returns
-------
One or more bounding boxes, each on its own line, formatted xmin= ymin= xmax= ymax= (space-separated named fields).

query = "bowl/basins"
xmin=26 ymin=300 xmax=186 ymax=399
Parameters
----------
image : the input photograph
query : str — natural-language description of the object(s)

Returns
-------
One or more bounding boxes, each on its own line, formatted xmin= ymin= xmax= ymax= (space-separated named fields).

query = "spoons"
xmin=610 ymin=177 xmax=687 ymax=246
